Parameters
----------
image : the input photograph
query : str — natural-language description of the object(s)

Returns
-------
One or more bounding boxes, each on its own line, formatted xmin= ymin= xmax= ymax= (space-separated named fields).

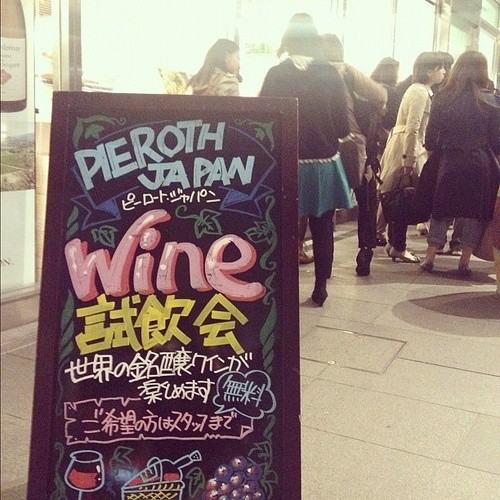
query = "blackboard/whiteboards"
xmin=27 ymin=90 xmax=304 ymax=500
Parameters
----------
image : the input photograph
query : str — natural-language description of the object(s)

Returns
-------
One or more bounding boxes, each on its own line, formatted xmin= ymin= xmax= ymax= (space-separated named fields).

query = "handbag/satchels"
xmin=380 ymin=174 xmax=431 ymax=225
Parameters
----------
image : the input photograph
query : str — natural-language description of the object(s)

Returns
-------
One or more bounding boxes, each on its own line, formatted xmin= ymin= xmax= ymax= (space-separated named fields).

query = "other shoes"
xmin=377 ymin=238 xmax=387 ymax=246
xmin=421 ymin=261 xmax=434 ymax=271
xmin=459 ymin=264 xmax=473 ymax=279
xmin=437 ymin=244 xmax=447 ymax=254
xmin=449 ymin=241 xmax=462 ymax=255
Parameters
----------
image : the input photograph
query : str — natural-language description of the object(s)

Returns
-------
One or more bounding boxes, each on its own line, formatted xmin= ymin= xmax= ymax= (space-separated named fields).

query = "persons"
xmin=259 ymin=12 xmax=350 ymax=307
xmin=189 ymin=39 xmax=240 ymax=97
xmin=398 ymin=52 xmax=454 ymax=105
xmin=378 ymin=53 xmax=446 ymax=264
xmin=315 ymin=27 xmax=388 ymax=276
xmin=414 ymin=164 xmax=500 ymax=300
xmin=420 ymin=49 xmax=500 ymax=279
xmin=370 ymin=57 xmax=400 ymax=246
xmin=294 ymin=215 xmax=315 ymax=264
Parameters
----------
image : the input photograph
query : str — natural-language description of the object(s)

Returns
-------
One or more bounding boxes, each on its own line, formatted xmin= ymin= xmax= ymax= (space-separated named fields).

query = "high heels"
xmin=416 ymin=223 xmax=429 ymax=236
xmin=390 ymin=247 xmax=421 ymax=263
xmin=385 ymin=243 xmax=415 ymax=257
xmin=311 ymin=289 xmax=328 ymax=308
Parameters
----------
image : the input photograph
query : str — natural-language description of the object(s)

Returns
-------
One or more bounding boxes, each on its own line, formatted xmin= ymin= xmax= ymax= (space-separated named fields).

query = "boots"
xmin=299 ymin=240 xmax=315 ymax=264
xmin=356 ymin=248 xmax=373 ymax=276
